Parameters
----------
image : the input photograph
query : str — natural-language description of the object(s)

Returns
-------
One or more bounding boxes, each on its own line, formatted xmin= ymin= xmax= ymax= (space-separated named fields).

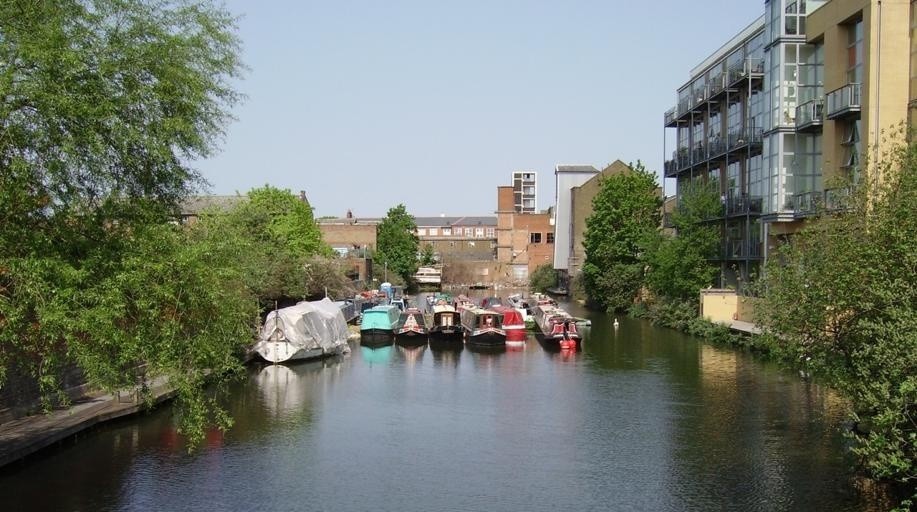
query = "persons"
xmin=486 ymin=316 xmax=492 ymax=328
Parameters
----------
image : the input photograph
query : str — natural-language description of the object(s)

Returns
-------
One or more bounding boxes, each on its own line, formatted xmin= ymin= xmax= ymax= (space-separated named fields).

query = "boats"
xmin=252 ymin=340 xmax=331 ymax=363
xmin=360 ymin=262 xmax=578 ymax=339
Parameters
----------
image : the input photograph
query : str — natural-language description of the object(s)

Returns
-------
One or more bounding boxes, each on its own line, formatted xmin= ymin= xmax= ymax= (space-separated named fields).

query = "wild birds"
xmin=613 ymin=318 xmax=619 ymax=326
